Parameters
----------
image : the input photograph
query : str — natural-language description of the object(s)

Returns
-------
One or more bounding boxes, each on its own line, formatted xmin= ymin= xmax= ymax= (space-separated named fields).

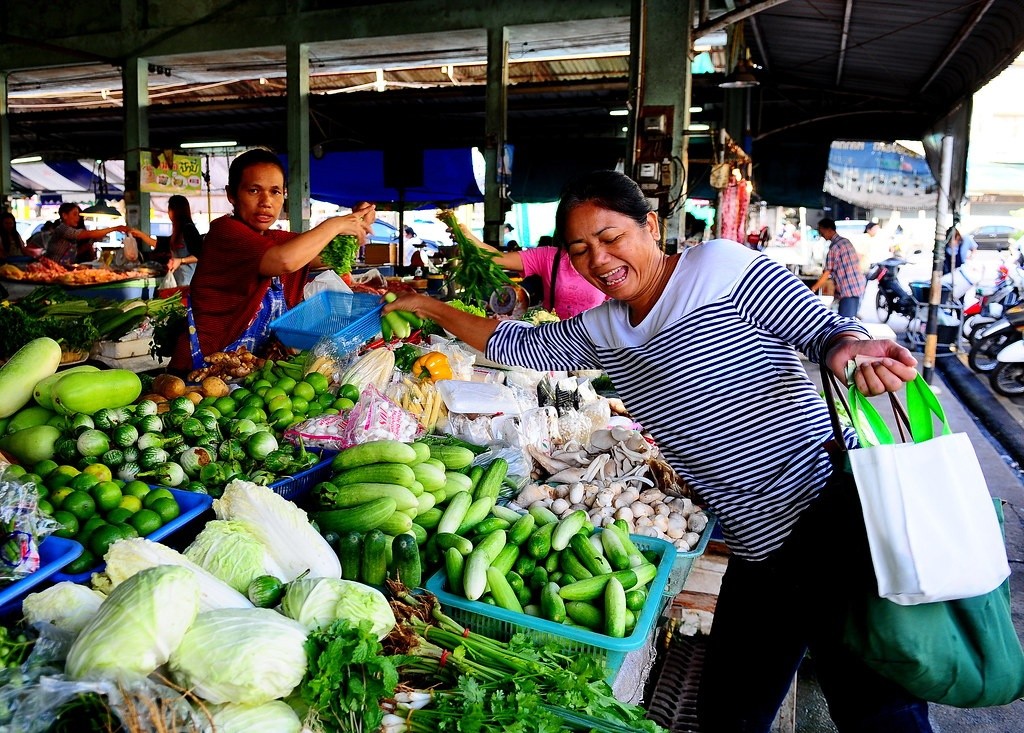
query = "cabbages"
xmin=18 ymin=479 xmax=396 ymax=733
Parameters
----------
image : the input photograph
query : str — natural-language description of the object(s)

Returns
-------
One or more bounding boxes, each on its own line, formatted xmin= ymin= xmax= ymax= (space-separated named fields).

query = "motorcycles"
xmin=870 ymin=250 xmax=923 ymax=324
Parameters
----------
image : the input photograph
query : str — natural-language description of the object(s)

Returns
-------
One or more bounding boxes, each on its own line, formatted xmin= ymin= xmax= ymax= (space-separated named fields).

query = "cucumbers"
xmin=297 ymin=441 xmax=658 ymax=640
xmin=380 ymin=291 xmax=424 ymax=342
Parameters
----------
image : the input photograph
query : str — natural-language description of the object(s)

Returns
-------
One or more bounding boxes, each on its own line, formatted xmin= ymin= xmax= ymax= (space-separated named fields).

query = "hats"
xmin=405 ymin=227 xmax=415 ymax=237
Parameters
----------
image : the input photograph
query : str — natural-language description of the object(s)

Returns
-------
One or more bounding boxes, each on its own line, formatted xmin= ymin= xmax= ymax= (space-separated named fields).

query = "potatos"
xmin=136 ymin=374 xmax=229 ymax=412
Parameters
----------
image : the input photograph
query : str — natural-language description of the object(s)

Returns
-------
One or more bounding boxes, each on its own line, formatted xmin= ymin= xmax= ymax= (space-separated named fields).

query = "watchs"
xmin=181 ymin=257 xmax=186 ymax=264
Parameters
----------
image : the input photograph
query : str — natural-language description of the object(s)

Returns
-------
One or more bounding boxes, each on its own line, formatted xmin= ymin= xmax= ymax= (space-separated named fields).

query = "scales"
xmin=488 ymin=269 xmax=530 ymax=320
xmin=101 ymin=247 xmax=120 ymax=256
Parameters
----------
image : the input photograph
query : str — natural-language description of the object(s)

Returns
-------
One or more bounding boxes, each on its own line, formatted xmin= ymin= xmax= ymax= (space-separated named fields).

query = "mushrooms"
xmin=515 ymin=426 xmax=709 ymax=553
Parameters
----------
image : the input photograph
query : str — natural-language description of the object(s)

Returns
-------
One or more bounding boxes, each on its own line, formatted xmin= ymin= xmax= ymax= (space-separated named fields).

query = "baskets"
xmin=0 ymin=444 xmax=342 ymax=616
xmin=268 ymin=288 xmax=388 ymax=361
xmin=425 ymin=479 xmax=724 ymax=694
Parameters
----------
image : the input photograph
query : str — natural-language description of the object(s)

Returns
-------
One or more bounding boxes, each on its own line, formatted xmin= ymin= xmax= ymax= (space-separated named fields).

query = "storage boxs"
xmin=426 ymin=479 xmax=718 ymax=688
xmin=365 ymin=243 xmax=398 ymax=264
xmin=100 ymin=339 xmax=155 ymax=359
xmin=909 ymin=282 xmax=950 ymax=304
xmin=0 ymin=442 xmax=334 ymax=613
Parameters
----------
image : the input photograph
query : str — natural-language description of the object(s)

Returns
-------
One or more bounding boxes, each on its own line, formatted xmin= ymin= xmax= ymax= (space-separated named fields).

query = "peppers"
xmin=362 ymin=329 xmax=453 ymax=385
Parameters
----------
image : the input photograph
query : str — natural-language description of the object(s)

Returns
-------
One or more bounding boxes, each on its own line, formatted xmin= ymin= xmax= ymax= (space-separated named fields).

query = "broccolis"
xmin=526 ymin=310 xmax=560 ymax=327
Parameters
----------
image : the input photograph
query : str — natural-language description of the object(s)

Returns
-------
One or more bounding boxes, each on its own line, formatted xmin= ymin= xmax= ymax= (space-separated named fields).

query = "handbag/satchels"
xmin=846 ymin=367 xmax=1011 ymax=606
xmin=203 ymin=281 xmax=293 ymax=367
xmin=303 ymin=269 xmax=354 ymax=320
xmin=157 ymin=269 xmax=178 ymax=290
xmin=844 ymin=366 xmax=1023 ymax=708
xmin=123 ymin=230 xmax=138 ymax=261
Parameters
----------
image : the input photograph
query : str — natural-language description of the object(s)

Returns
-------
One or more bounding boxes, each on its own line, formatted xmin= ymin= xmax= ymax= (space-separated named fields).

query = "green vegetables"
xmin=284 ymin=584 xmax=673 ymax=733
xmin=422 ymin=298 xmax=485 ymax=336
xmin=435 ymin=210 xmax=520 ymax=308
xmin=318 ymin=232 xmax=360 ymax=276
xmin=0 ymin=283 xmax=191 ymax=364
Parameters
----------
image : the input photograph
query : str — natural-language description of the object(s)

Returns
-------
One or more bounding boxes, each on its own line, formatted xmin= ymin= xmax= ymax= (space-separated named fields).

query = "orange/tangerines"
xmin=2 ymin=460 xmax=180 ymax=574
xmin=200 ymin=371 xmax=361 ymax=444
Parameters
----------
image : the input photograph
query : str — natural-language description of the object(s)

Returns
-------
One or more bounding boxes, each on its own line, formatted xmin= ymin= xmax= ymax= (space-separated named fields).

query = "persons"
xmin=402 ymin=224 xmax=423 ymax=266
xmin=809 ymin=217 xmax=867 ymax=317
xmin=856 ymin=222 xmax=879 ymax=320
xmin=445 ymin=223 xmax=612 ymax=322
xmin=380 ymin=171 xmax=937 ymax=732
xmin=165 ymin=148 xmax=378 ymax=374
xmin=0 ymin=195 xmax=203 ymax=286
xmin=941 ymin=225 xmax=978 ymax=305
xmin=503 ymin=223 xmax=514 ymax=246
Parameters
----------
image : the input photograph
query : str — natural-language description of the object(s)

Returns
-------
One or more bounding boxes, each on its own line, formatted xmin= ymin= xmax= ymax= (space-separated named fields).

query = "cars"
xmin=16 ymin=219 xmax=48 ymax=245
xmin=314 ymin=212 xmax=444 ymax=251
xmin=114 ymin=219 xmax=172 ymax=242
xmin=968 ymin=226 xmax=1015 ymax=252
xmin=820 ymin=221 xmax=869 ymax=245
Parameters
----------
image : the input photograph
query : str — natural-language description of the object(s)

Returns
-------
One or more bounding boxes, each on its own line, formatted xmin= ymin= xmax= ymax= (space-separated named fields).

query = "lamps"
xmin=79 ymin=176 xmax=122 ymax=219
xmin=714 ymin=45 xmax=760 ymax=88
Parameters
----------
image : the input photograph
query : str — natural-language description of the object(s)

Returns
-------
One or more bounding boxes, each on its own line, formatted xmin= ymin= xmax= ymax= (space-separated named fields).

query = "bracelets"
xmin=810 ymin=287 xmax=816 ymax=292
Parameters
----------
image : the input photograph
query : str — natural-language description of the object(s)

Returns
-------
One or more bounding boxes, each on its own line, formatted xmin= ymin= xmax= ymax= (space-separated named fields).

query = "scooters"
xmin=961 ymin=265 xmax=1024 ymax=397
